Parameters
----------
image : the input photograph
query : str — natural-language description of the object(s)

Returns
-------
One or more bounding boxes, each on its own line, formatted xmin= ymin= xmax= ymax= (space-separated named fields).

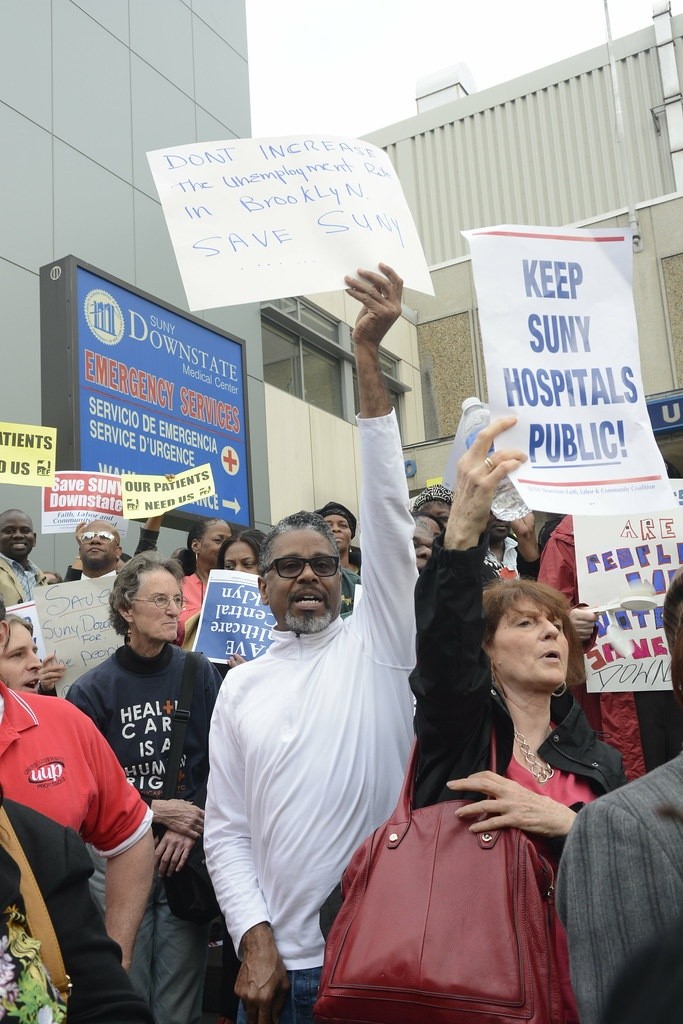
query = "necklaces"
xmin=512 ymin=723 xmax=554 ymax=784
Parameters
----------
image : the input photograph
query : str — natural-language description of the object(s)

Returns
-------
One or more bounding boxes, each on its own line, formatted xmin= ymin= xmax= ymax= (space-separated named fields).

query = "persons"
xmin=0 ymin=485 xmax=683 ymax=1024
xmin=201 ymin=264 xmax=420 ymax=1024
xmin=408 ymin=417 xmax=631 ymax=1024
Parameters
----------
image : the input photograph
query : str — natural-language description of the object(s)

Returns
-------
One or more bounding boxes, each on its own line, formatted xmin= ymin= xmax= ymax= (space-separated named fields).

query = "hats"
xmin=413 ymin=484 xmax=453 ymax=514
xmin=314 ymin=501 xmax=356 ymax=540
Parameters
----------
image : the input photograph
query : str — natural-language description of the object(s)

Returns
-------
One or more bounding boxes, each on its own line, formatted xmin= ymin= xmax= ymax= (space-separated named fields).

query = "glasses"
xmin=263 ymin=555 xmax=340 ymax=578
xmin=133 ymin=596 xmax=188 ymax=610
xmin=79 ymin=531 xmax=119 ymax=546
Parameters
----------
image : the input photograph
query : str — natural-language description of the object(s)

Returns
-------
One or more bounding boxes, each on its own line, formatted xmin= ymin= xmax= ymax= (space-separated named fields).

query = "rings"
xmin=484 ymin=458 xmax=494 ymax=471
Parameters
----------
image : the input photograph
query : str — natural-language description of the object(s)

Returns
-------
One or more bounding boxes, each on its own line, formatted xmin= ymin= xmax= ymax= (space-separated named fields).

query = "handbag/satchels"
xmin=311 ymin=719 xmax=581 ymax=1024
xmin=157 ymin=825 xmax=223 ymax=923
